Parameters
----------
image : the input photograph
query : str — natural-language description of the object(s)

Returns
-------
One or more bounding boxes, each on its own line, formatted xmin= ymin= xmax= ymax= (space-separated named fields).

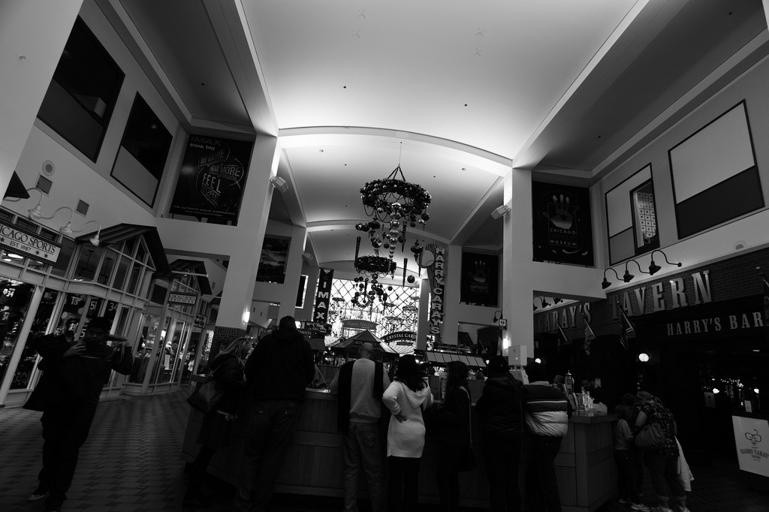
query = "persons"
xmin=25 ymin=317 xmax=133 ymax=509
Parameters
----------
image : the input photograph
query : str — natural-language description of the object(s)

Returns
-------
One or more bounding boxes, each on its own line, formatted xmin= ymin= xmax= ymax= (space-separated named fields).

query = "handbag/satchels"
xmin=673 ymin=434 xmax=695 ymax=492
xmin=633 ymin=422 xmax=666 ymax=451
xmin=186 ymin=377 xmax=225 ymax=415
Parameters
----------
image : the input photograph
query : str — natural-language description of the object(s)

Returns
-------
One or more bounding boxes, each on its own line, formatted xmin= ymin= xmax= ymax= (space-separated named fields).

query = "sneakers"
xmin=25 ymin=490 xmax=48 ymax=501
xmin=619 ymin=495 xmax=691 ymax=512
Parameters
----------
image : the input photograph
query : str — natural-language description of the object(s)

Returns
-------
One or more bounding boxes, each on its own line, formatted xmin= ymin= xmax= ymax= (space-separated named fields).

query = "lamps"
xmin=601 ymin=249 xmax=681 ymax=291
xmin=353 ymin=137 xmax=433 ymax=315
xmin=4 ymin=187 xmax=102 ymax=248
